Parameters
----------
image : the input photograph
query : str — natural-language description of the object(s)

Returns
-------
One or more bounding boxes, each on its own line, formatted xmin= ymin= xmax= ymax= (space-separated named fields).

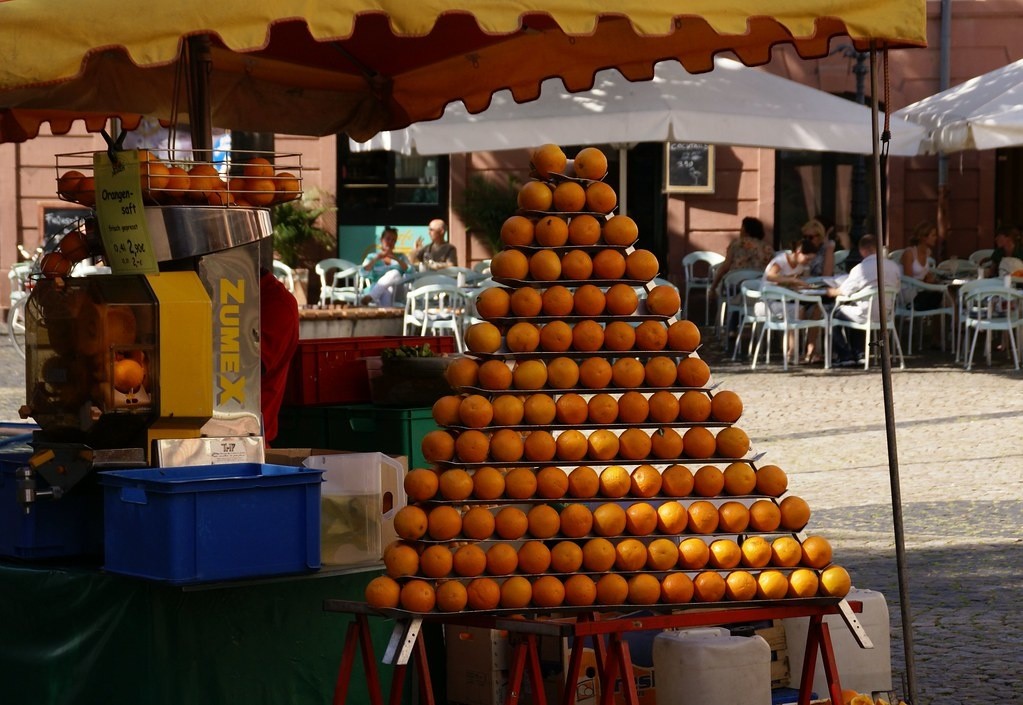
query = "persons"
xmin=410 ymin=219 xmax=458 ymax=273
xmin=707 ymin=215 xmax=1022 ymax=368
xmin=358 ymin=228 xmax=414 ymax=307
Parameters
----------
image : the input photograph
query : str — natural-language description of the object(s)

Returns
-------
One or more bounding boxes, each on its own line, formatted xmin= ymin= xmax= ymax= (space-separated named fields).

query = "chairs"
xmin=272 ymin=259 xmax=497 ymax=355
xmin=682 ymin=250 xmax=1023 ymax=373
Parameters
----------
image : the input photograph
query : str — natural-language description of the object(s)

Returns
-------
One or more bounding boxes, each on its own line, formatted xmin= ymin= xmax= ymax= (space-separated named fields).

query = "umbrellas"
xmin=0 ymin=0 xmax=1022 ymax=170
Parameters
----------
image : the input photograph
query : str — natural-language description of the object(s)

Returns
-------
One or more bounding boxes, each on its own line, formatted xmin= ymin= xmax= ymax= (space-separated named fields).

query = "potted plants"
xmin=375 ymin=344 xmax=452 ymax=407
xmin=268 ymin=189 xmax=339 ymax=309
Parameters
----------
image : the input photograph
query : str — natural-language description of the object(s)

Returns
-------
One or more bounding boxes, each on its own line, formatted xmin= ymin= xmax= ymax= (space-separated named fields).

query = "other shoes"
xmin=833 ymin=356 xmax=856 ymax=366
xmin=362 ymin=297 xmax=373 ymax=305
xmin=787 ymin=353 xmax=808 ymax=365
xmin=855 ymin=352 xmax=865 ymax=364
xmin=804 ymin=354 xmax=824 ymax=365
xmin=930 ymin=340 xmax=951 ymax=351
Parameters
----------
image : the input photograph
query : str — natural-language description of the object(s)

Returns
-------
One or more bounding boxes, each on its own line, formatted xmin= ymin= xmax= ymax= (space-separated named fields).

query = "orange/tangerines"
xmin=31 ymin=150 xmax=299 ymax=428
xmin=366 ymin=144 xmax=852 ymax=611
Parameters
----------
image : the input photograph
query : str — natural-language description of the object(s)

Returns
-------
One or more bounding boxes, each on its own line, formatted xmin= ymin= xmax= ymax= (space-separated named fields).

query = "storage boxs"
xmin=281 ymin=336 xmax=456 ymax=406
xmin=98 ymin=462 xmax=325 ymax=584
xmin=269 ymin=405 xmax=447 ymax=471
xmin=263 ymin=447 xmax=410 ymax=566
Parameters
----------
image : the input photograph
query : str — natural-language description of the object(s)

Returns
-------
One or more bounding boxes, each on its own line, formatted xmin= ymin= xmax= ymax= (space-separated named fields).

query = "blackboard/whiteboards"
xmin=664 ymin=142 xmax=716 ymax=194
xmin=36 ymin=199 xmax=94 ymax=257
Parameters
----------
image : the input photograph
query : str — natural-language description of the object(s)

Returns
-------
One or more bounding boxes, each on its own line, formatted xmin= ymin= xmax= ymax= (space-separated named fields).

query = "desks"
xmin=0 ymin=564 xmax=400 ymax=705
